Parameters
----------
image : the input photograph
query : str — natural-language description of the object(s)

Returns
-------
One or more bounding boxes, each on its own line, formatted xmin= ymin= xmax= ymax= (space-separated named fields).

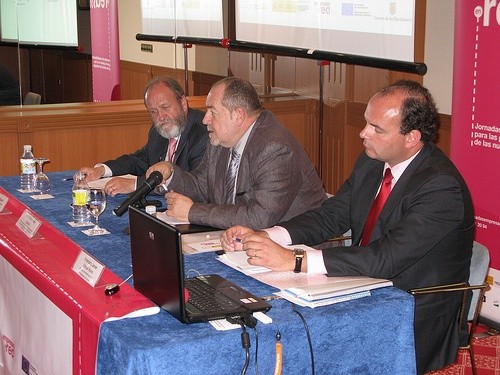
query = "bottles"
xmin=145 ymin=205 xmax=157 ymax=218
xmin=71 ymin=172 xmax=91 ymax=225
xmin=20 ymin=144 xmax=36 ymax=191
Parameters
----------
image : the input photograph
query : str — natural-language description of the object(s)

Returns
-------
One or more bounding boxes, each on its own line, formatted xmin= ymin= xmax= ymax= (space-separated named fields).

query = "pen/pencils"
xmin=162 ymin=184 xmax=169 ymax=193
xmin=62 ymin=178 xmax=73 ymax=181
xmin=232 ymin=237 xmax=241 ymax=242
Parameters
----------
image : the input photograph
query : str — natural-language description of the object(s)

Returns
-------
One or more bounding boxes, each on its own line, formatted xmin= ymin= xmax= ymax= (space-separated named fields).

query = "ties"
xmin=360 ymin=169 xmax=394 ymax=246
xmin=166 ymin=138 xmax=177 ymax=164
xmin=223 ymin=147 xmax=241 ymax=204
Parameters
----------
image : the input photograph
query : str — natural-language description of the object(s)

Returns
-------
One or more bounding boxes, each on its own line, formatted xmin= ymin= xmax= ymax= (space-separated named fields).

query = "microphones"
xmin=112 ymin=170 xmax=163 ymax=217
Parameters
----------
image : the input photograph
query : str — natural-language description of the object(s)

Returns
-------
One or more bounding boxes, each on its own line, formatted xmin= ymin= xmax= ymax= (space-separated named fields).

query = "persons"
xmin=145 ymin=76 xmax=345 ymax=250
xmin=220 ymin=79 xmax=475 ymax=371
xmin=73 ymin=78 xmax=210 ymax=196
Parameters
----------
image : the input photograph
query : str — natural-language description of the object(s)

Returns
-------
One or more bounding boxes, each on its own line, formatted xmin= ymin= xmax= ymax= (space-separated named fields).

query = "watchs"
xmin=292 ymin=248 xmax=305 ymax=273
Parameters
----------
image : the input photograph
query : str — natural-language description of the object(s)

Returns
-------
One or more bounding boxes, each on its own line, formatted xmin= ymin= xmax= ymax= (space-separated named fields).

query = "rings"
xmin=255 ymin=250 xmax=256 ymax=257
xmin=111 ymin=187 xmax=114 ymax=191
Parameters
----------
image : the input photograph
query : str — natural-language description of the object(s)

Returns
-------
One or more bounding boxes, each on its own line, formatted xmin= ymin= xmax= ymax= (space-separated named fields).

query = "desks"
xmin=0 ymin=170 xmax=416 ymax=375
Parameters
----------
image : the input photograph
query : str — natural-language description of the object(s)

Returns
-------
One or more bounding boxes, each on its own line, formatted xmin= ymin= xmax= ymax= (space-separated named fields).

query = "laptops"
xmin=127 ymin=204 xmax=272 ymax=325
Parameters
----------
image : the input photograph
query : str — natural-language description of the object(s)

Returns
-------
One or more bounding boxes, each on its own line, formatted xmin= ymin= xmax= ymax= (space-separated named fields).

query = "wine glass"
xmin=31 ymin=157 xmax=50 ymax=198
xmin=85 ymin=187 xmax=107 ymax=236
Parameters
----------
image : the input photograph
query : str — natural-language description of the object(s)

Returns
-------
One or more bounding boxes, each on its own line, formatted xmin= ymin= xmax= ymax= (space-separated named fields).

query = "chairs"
xmin=312 ymin=192 xmax=491 ymax=375
xmin=24 ymin=92 xmax=41 ymax=105
